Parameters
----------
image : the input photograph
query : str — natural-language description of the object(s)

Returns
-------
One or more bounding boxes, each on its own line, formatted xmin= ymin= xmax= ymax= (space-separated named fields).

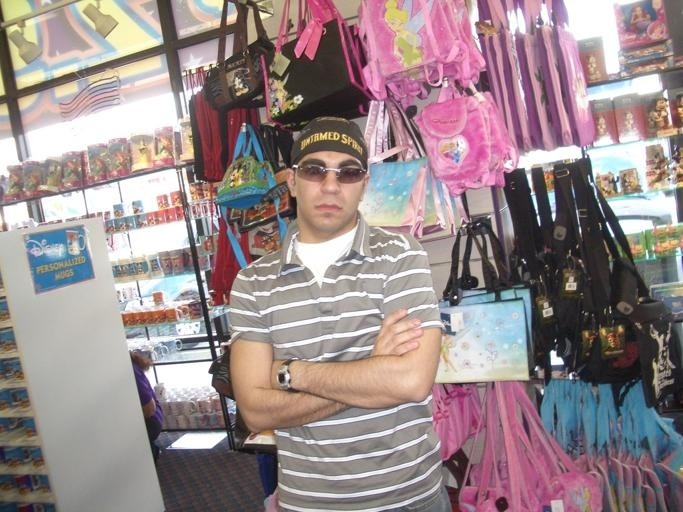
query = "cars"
xmin=522 ymin=157 xmax=678 ymax=230
xmin=124 ymin=240 xmax=213 ymax=354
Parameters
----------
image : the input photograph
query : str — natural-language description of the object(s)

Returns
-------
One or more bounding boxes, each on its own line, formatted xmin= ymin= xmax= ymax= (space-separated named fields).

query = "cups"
xmin=66 ymin=230 xmax=84 ymax=256
xmin=153 ymin=383 xmax=238 ymax=428
xmin=106 ymin=182 xmax=208 ymax=279
xmin=0 ymin=296 xmax=58 ymax=510
xmin=134 ymin=341 xmax=184 ymax=362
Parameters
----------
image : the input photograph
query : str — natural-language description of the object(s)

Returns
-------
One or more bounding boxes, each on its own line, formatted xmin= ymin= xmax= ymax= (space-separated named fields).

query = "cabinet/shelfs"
xmin=1 ymin=154 xmax=242 ymax=454
xmin=1 ymin=215 xmax=170 ymax=511
xmin=577 ymin=66 xmax=683 ymax=265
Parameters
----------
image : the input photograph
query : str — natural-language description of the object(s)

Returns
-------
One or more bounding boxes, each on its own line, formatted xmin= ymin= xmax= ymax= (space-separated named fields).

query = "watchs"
xmin=277 ymin=357 xmax=300 ymax=394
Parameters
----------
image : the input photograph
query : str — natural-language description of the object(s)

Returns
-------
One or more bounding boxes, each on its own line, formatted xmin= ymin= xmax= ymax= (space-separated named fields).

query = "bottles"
xmin=120 ymin=292 xmax=207 ymax=326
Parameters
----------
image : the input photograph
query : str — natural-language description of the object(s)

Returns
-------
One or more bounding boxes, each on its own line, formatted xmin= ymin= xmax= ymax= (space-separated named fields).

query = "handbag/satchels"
xmin=358 ymin=1 xmax=597 ymax=242
xmin=264 ymin=0 xmax=374 ymax=120
xmin=175 ymin=0 xmax=297 ymax=313
xmin=432 ymin=217 xmax=682 ymax=512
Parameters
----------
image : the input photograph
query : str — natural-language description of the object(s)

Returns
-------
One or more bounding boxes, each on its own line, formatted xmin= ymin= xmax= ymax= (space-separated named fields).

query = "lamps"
xmin=0 ymin=0 xmax=120 ymax=64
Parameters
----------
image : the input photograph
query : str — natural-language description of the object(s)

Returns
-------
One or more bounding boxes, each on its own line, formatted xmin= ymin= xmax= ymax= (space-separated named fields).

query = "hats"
xmin=288 ymin=118 xmax=370 ymax=162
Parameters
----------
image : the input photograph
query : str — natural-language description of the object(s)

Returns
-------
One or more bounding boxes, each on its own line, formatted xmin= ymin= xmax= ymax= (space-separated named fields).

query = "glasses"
xmin=291 ymin=162 xmax=369 ymax=183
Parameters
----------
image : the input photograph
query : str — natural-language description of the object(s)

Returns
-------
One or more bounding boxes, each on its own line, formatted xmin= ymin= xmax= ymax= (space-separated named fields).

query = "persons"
xmin=228 ymin=113 xmax=454 ymax=510
xmin=212 ymin=351 xmax=279 ymax=497
xmin=130 ymin=352 xmax=164 ymax=462
